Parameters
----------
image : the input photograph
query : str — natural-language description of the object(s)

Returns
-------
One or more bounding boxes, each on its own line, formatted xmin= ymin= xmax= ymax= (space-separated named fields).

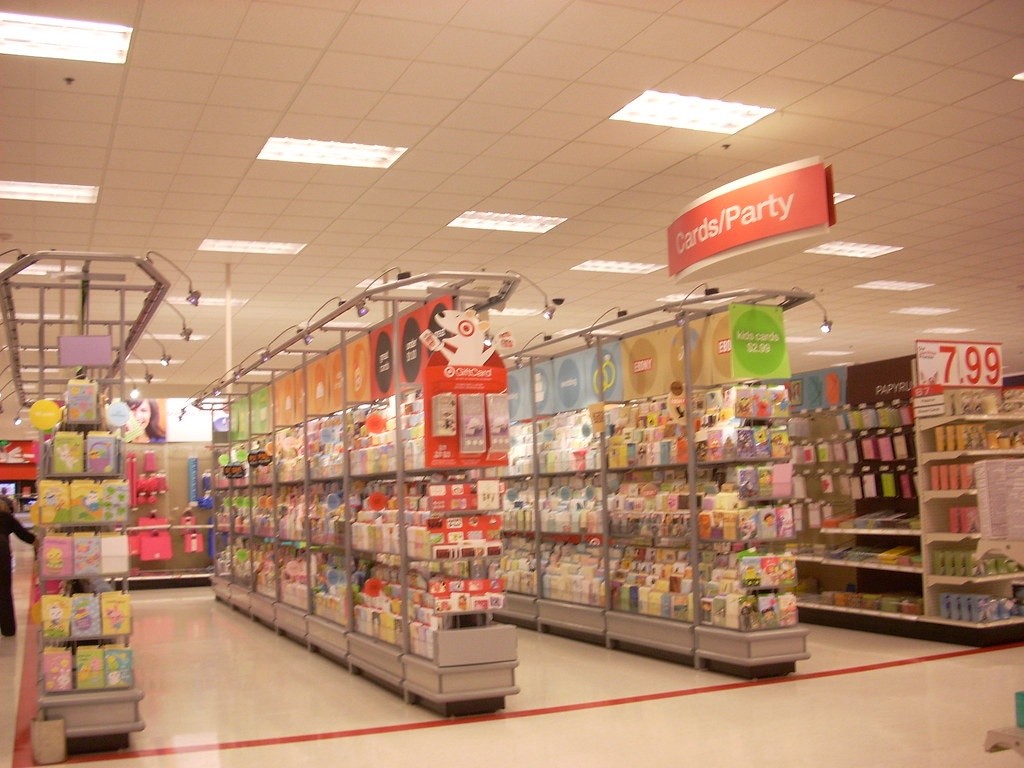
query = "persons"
xmin=0 ymin=488 xmax=40 ymax=636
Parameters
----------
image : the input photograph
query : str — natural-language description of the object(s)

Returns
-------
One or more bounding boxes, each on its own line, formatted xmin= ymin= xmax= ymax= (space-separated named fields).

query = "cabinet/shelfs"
xmin=788 ymin=353 xmax=1024 ymax=648
xmin=497 ymin=281 xmax=832 ymax=680
xmin=0 ymin=250 xmax=202 ymax=755
xmin=209 ymin=270 xmax=530 ymax=718
